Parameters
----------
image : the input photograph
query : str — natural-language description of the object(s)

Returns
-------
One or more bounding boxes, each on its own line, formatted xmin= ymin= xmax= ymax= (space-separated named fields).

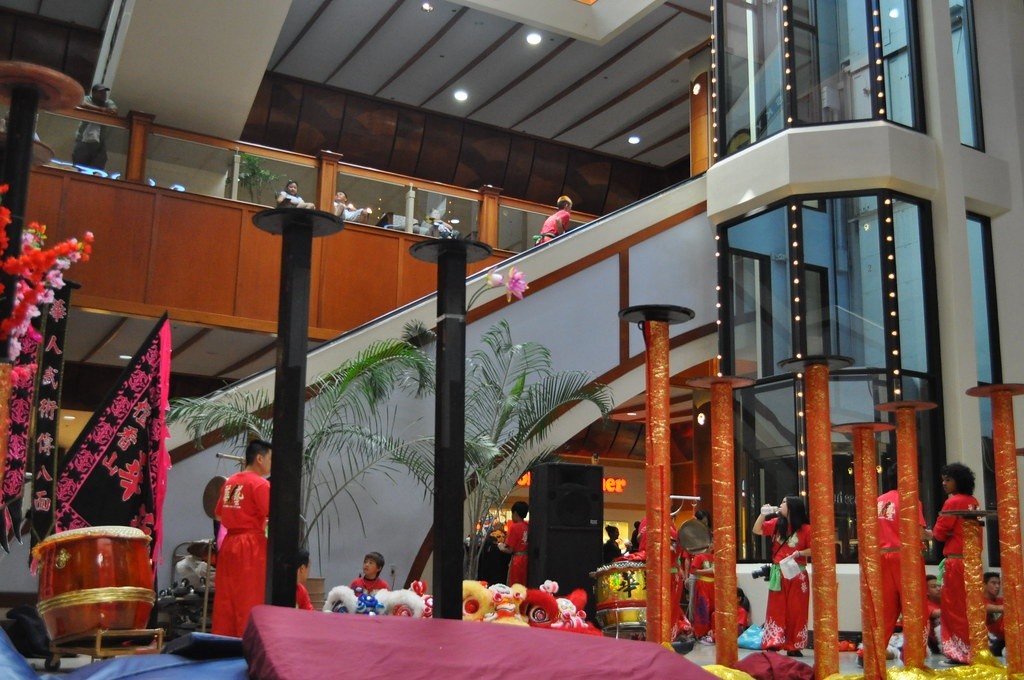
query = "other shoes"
xmin=939 ymin=657 xmax=962 ymax=667
xmin=857 ymin=655 xmax=863 ymax=666
xmin=787 ymin=650 xmax=803 ymax=657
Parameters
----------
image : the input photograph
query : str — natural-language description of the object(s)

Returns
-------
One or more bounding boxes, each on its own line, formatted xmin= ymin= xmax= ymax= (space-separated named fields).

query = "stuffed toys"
xmin=322 ymin=579 xmax=603 ymax=637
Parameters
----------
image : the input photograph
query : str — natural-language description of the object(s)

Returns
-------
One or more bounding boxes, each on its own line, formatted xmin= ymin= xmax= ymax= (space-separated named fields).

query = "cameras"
xmin=752 ymin=566 xmax=771 ymax=581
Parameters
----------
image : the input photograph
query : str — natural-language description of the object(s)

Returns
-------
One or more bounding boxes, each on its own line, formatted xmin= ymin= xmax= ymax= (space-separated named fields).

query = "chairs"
xmin=275 ymin=189 xmax=303 ymax=206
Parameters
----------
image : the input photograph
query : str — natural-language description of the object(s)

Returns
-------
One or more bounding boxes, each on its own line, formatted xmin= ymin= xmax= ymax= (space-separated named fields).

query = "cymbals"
xmin=678 ymin=518 xmax=710 ymax=555
xmin=202 ymin=475 xmax=230 ymax=521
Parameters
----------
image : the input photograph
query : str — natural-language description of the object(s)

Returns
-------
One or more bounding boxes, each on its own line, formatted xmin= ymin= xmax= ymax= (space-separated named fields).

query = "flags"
xmin=52 ymin=315 xmax=172 ymax=570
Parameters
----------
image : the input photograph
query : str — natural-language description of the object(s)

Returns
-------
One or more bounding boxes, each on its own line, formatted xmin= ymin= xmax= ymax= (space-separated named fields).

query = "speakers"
xmin=526 ymin=462 xmax=603 ymax=629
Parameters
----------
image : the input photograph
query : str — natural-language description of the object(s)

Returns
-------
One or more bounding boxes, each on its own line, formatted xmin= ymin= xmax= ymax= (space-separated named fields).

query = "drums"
xmin=593 ymin=562 xmax=647 ymax=633
xmin=32 ymin=525 xmax=156 ymax=642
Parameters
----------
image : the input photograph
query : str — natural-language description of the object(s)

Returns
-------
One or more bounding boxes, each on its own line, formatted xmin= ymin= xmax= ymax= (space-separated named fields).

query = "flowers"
xmin=0 ymin=184 xmax=95 ymax=384
xmin=468 ymin=265 xmax=531 ymax=315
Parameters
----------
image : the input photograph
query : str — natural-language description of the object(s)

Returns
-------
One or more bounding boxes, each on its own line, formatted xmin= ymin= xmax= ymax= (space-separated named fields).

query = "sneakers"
xmin=702 ymin=636 xmax=714 ymax=645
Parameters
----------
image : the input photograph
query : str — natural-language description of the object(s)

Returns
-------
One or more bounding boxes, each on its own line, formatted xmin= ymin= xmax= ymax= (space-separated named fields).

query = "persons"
xmin=334 ymin=188 xmax=369 ymax=224
xmin=351 ymin=552 xmax=390 ymax=595
xmin=70 ymin=83 xmax=118 ymax=176
xmin=213 ymin=440 xmax=271 ymax=637
xmin=919 ymin=574 xmax=946 ymax=653
xmin=500 ymin=502 xmax=530 ymax=587
xmin=295 ymin=547 xmax=318 ymax=611
xmin=428 ymin=209 xmax=453 ymax=239
xmin=933 ymin=461 xmax=984 ymax=667
xmin=983 ymin=571 xmax=1005 ymax=657
xmin=277 ymin=178 xmax=317 ymax=210
xmin=859 ymin=464 xmax=933 ymax=662
xmin=533 ymin=195 xmax=573 ymax=247
xmin=753 ymin=494 xmax=811 ymax=657
xmin=604 ymin=511 xmax=751 ymax=645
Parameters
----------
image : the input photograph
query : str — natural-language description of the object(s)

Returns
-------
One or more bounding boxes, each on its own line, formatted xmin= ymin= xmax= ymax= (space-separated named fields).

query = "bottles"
xmin=761 ymin=506 xmax=781 ymax=514
